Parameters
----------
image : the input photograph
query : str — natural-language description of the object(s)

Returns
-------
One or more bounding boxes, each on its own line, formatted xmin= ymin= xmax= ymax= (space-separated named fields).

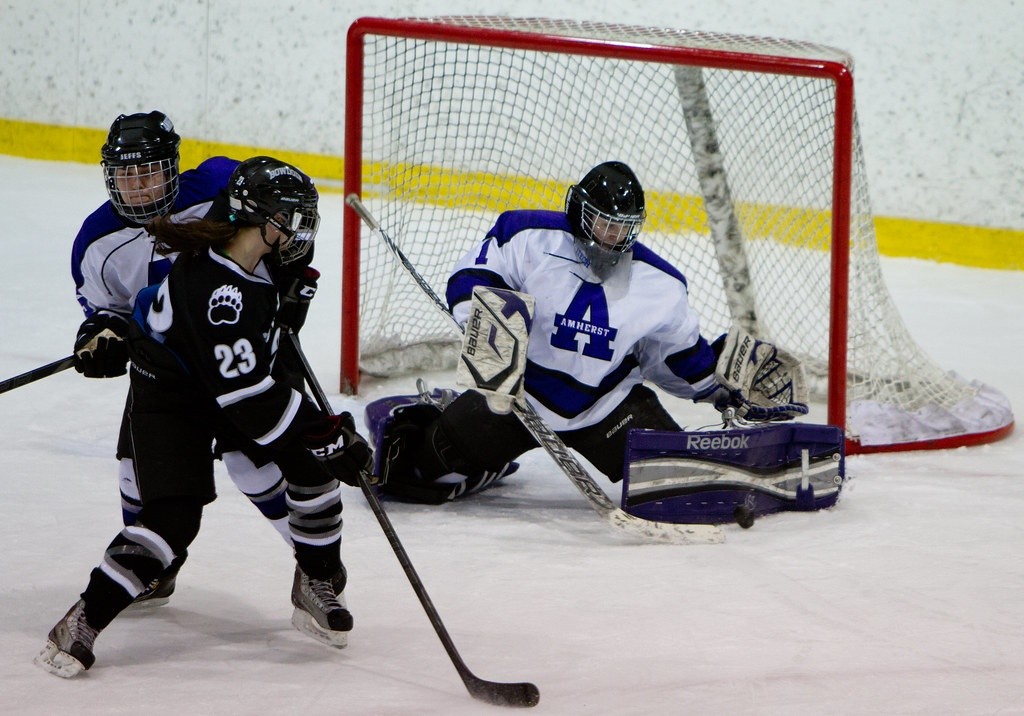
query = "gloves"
xmin=73 ymin=313 xmax=130 ymax=378
xmin=299 ymin=411 xmax=374 ymax=488
xmin=273 ymin=266 xmax=320 ymax=336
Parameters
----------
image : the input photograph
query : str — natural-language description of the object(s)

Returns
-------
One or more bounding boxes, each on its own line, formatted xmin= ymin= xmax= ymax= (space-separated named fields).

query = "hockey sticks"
xmin=1 ymin=353 xmax=78 ymax=396
xmin=344 ymin=192 xmax=720 ymax=544
xmin=283 ymin=330 xmax=542 ymax=711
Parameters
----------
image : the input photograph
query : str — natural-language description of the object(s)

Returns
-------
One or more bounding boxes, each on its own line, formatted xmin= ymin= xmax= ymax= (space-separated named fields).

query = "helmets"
xmin=101 ymin=110 xmax=181 ymax=227
xmin=228 ymin=154 xmax=320 ymax=266
xmin=570 ymin=161 xmax=647 ymax=279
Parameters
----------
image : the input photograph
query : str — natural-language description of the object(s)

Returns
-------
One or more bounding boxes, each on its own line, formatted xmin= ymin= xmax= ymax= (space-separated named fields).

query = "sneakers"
xmin=35 ymin=598 xmax=99 ymax=677
xmin=124 ymin=549 xmax=188 ymax=614
xmin=290 ymin=561 xmax=353 ymax=649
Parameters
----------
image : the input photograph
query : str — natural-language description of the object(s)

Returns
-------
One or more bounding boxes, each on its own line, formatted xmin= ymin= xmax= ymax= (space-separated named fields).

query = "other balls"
xmin=734 ymin=503 xmax=754 ymax=530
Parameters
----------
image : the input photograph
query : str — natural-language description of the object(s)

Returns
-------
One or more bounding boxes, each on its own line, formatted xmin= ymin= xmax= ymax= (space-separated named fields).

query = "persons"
xmin=72 ymin=111 xmax=347 ymax=612
xmin=31 ymin=155 xmax=374 ymax=679
xmin=364 ymin=158 xmax=845 ymax=526
